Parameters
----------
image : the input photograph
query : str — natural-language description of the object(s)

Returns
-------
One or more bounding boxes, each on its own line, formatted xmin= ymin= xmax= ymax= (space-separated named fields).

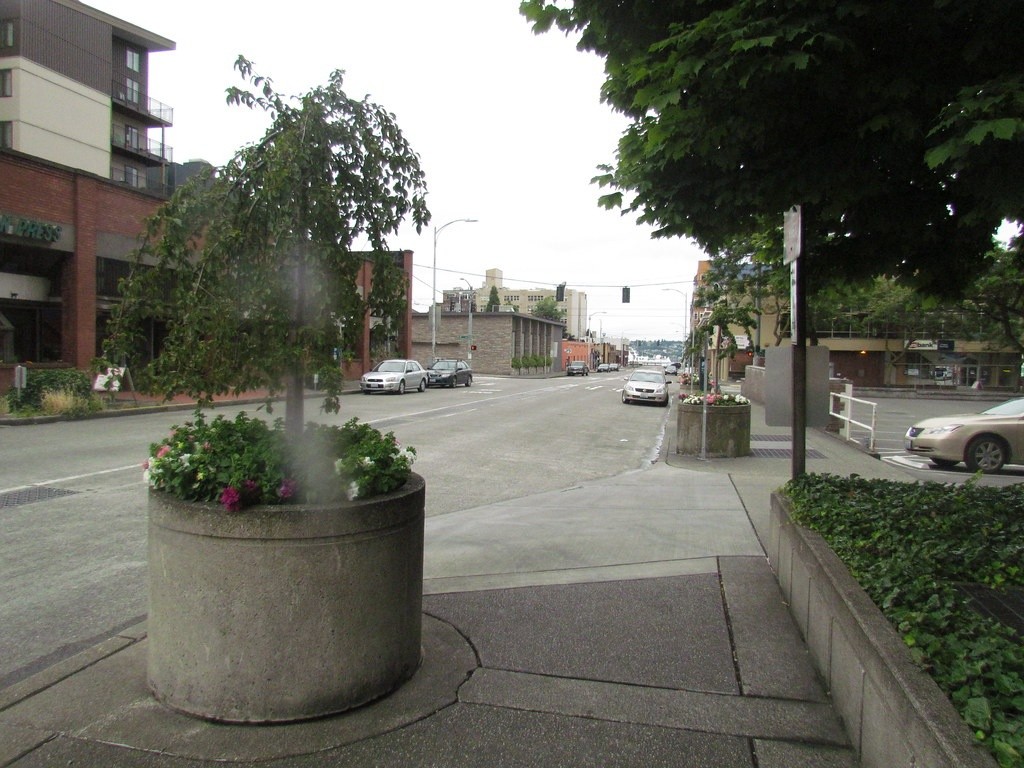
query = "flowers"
xmin=679 ymin=370 xmax=752 ymax=407
xmin=140 ymin=405 xmax=419 ymax=517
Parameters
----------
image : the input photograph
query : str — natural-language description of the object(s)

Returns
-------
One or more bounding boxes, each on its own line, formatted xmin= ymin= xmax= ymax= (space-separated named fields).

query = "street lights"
xmin=460 ymin=275 xmax=473 ymax=369
xmin=660 ymin=287 xmax=688 ymax=377
xmin=431 ymin=217 xmax=479 ymax=368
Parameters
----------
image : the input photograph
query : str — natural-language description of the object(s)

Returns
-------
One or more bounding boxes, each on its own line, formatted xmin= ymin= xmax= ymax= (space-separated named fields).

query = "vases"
xmin=674 ymin=400 xmax=753 ymax=458
xmin=682 ymin=384 xmax=701 ymax=394
xmin=139 ymin=468 xmax=430 ymax=722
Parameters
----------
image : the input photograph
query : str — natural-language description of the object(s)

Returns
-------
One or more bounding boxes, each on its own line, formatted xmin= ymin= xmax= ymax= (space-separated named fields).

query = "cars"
xmin=597 ymin=363 xmax=609 ymax=372
xmin=425 ymin=358 xmax=474 ymax=388
xmin=566 ymin=361 xmax=589 ymax=376
xmin=901 ymin=395 xmax=1024 ymax=476
xmin=622 ymin=368 xmax=672 ymax=407
xmin=359 ymin=359 xmax=429 ymax=394
xmin=664 ymin=365 xmax=677 ymax=376
xmin=609 ymin=363 xmax=618 ymax=371
